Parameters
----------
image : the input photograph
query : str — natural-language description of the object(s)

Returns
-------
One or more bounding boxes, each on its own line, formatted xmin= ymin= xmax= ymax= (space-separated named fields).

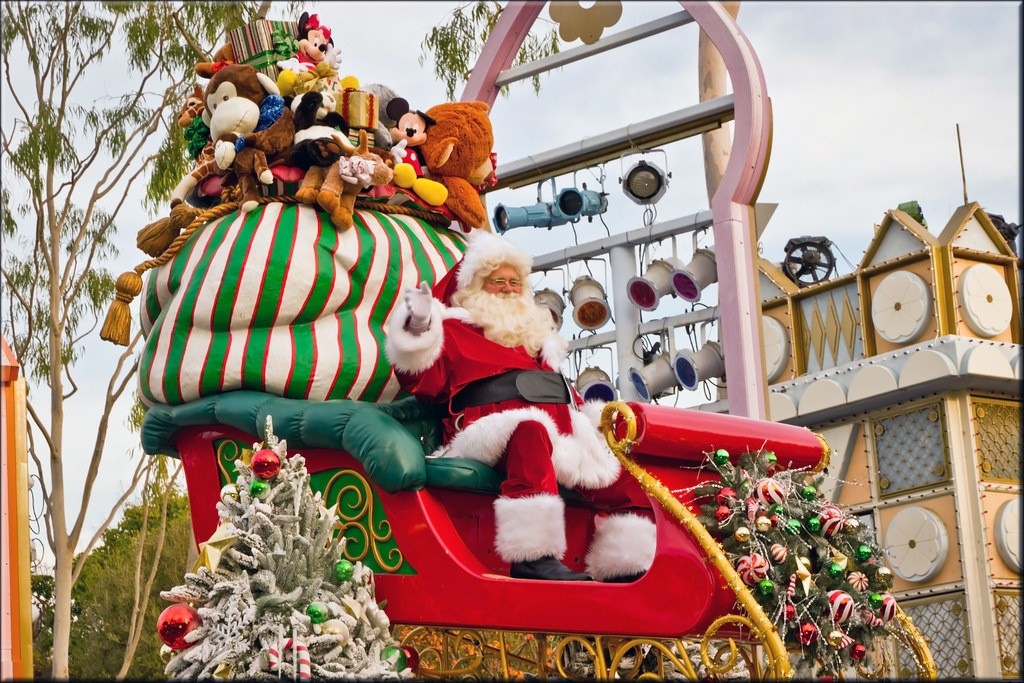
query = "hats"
xmin=456 ymin=232 xmax=532 ymax=289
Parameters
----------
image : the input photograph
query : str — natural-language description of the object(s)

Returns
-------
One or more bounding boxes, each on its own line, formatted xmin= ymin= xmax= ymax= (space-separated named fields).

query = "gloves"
xmin=404 ymin=280 xmax=432 ymax=332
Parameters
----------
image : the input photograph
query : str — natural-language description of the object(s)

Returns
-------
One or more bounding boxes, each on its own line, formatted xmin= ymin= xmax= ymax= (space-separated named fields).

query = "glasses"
xmin=485 ymin=279 xmax=523 ymax=287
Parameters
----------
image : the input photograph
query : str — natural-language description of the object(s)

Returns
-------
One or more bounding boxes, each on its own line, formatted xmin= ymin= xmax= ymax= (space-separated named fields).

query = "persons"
xmin=383 ymin=232 xmax=657 ymax=583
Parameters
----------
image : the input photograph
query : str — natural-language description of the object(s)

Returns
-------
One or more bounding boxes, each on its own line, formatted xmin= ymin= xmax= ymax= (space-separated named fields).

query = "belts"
xmin=444 ymin=371 xmax=582 ymax=417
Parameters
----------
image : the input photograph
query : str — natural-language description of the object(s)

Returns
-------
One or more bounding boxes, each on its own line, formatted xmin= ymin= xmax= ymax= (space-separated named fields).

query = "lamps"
xmin=531 ymin=224 xmax=725 ymax=403
xmin=493 ymin=175 xmax=567 ymax=234
xmin=618 ymin=149 xmax=673 ymax=205
xmin=556 ymin=165 xmax=609 ymax=220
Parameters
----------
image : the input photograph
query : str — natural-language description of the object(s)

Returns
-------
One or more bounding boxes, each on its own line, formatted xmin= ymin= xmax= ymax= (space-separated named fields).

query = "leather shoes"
xmin=510 ymin=555 xmax=594 ymax=581
xmin=603 ymin=569 xmax=647 ymax=582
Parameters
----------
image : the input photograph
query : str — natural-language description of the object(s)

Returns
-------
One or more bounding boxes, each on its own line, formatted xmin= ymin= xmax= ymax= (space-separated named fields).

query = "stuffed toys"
xmin=178 ymin=12 xmax=495 ymax=231
